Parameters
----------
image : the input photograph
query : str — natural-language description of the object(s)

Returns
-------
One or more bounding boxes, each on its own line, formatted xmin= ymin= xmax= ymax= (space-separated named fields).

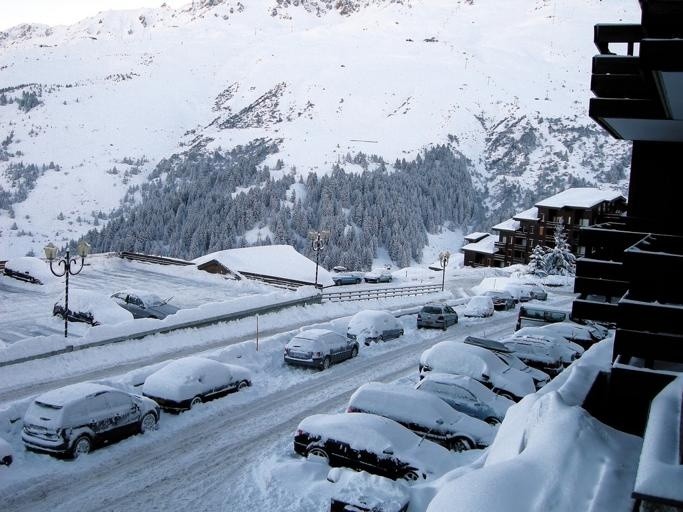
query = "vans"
xmin=513 ymin=304 xmax=578 ymax=333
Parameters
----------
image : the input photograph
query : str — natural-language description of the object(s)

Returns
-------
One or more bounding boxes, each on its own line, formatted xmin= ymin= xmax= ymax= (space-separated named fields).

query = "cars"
xmin=463 ymin=296 xmax=495 ymax=318
xmin=3 ymin=256 xmax=44 ymax=285
xmin=507 ymin=283 xmax=548 ymax=302
xmin=347 ymin=312 xmax=405 ymax=347
xmin=416 ymin=303 xmax=459 ymax=331
xmin=53 ymin=289 xmax=182 ymax=327
xmin=332 ymin=268 xmax=393 ymax=285
xmin=282 ymin=329 xmax=359 ymax=371
xmin=294 ymin=412 xmax=456 ymax=485
xmin=504 ymin=322 xmax=608 ymax=372
xmin=484 ymin=289 xmax=516 ymax=311
xmin=415 ymin=373 xmax=515 ymax=425
xmin=464 ymin=335 xmax=553 ymax=391
xmin=346 ymin=382 xmax=493 ymax=453
xmin=417 ymin=340 xmax=536 ymax=403
xmin=0 ymin=357 xmax=251 ymax=468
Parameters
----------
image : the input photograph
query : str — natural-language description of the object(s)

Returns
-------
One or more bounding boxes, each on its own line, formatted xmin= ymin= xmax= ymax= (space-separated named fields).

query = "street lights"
xmin=438 ymin=251 xmax=451 ymax=288
xmin=43 ymin=240 xmax=90 ymax=337
xmin=309 ymin=228 xmax=330 ymax=289
